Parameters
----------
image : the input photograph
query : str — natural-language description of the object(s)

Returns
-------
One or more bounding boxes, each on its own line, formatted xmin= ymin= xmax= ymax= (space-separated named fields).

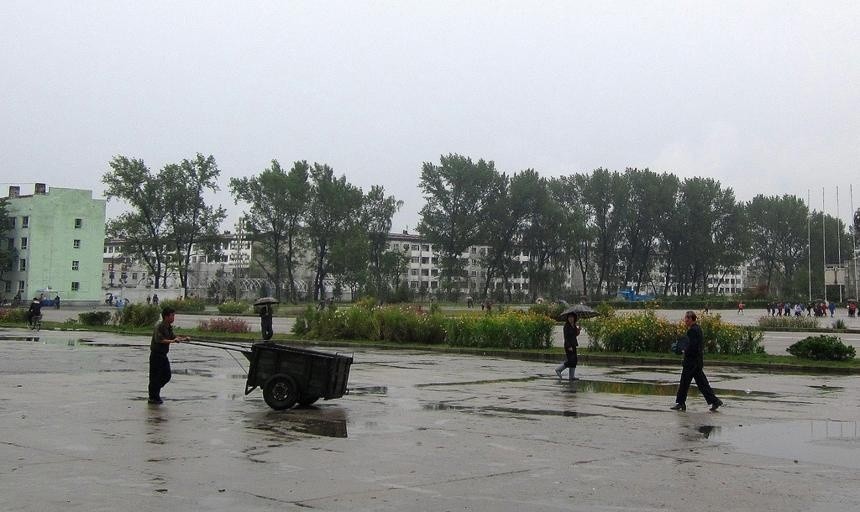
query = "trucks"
xmin=619 ymin=287 xmax=657 ymax=302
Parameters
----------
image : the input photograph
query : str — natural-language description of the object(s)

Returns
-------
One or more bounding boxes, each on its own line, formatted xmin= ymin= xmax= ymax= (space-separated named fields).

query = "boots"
xmin=569 ymin=367 xmax=579 ymax=379
xmin=555 ymin=364 xmax=565 ymax=378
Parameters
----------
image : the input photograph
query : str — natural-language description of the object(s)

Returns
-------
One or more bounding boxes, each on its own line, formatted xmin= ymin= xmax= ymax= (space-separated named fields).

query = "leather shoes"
xmin=671 ymin=403 xmax=685 ymax=410
xmin=148 ymin=396 xmax=162 ymax=403
xmin=710 ymin=400 xmax=722 ymax=410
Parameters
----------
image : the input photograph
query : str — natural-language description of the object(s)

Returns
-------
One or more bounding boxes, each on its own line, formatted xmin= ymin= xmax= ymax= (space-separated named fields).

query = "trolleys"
xmin=175 ymin=337 xmax=353 ymax=410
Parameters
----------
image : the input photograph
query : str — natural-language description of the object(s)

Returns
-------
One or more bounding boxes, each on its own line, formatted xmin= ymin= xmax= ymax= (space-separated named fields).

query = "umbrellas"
xmin=559 ymin=302 xmax=601 ymax=318
xmin=253 ymin=296 xmax=279 ymax=316
xmin=105 ymin=293 xmax=112 ymax=295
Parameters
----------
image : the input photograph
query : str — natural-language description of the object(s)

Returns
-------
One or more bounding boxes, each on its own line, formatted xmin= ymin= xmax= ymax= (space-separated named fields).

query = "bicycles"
xmin=26 ymin=310 xmax=42 ymax=331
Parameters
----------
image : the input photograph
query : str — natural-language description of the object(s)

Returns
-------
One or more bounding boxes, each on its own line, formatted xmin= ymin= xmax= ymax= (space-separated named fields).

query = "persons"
xmin=739 ymin=300 xmax=860 ymax=318
xmin=109 ymin=294 xmax=113 ymax=304
xmin=147 ymin=294 xmax=159 ymax=306
xmin=148 ymin=306 xmax=192 ymax=404
xmin=704 ymin=302 xmax=708 ymax=314
xmin=555 ymin=312 xmax=582 ymax=381
xmin=670 ymin=310 xmax=723 ymax=412
xmin=258 ymin=303 xmax=274 ymax=340
xmin=55 ymin=296 xmax=61 ymax=310
xmin=2 ymin=295 xmax=45 ymax=307
xmin=28 ymin=297 xmax=42 ymax=325
